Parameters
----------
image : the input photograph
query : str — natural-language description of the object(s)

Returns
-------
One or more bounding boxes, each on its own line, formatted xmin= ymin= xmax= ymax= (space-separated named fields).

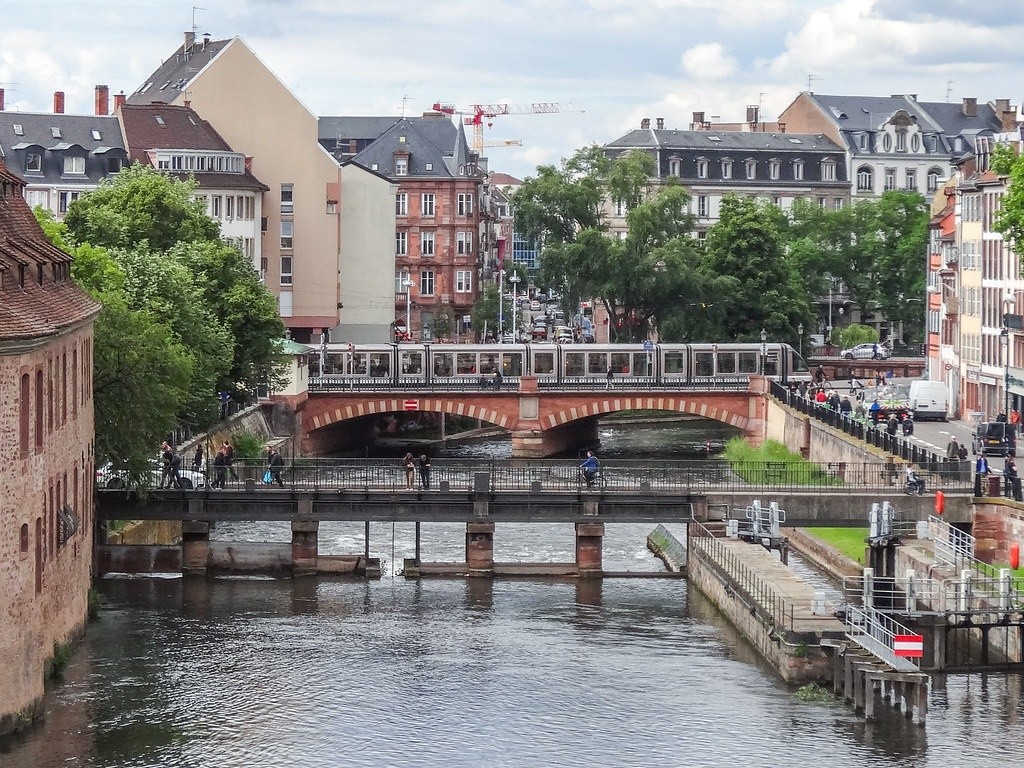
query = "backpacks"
xmin=593 ymin=457 xmax=601 ymax=466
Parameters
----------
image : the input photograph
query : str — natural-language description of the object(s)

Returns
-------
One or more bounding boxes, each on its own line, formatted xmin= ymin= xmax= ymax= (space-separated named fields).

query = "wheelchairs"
xmin=903 ymin=475 xmax=920 ymax=496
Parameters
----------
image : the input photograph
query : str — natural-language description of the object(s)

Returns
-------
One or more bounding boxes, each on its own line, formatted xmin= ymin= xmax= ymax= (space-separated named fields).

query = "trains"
xmin=305 ymin=343 xmax=815 ymax=388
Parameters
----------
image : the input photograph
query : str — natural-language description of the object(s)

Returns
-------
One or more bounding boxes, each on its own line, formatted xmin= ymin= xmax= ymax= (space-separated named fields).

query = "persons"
xmin=907 ymin=464 xmax=930 ymax=496
xmin=946 ymin=435 xmax=968 ymax=461
xmin=872 ymin=342 xmax=880 ymax=360
xmin=605 ymin=368 xmax=614 ymax=389
xmin=976 ymin=453 xmax=993 ymax=475
xmin=996 ymin=407 xmax=1020 ymax=424
xmin=266 ymin=445 xmax=285 ymax=487
xmin=788 ymin=363 xmax=899 ymax=435
xmin=1005 ymin=449 xmax=1018 ymax=477
xmin=401 ymin=452 xmax=432 ymax=492
xmin=480 ymin=368 xmax=502 ymax=389
xmin=579 ymin=451 xmax=598 ymax=491
xmin=157 ymin=440 xmax=239 ymax=491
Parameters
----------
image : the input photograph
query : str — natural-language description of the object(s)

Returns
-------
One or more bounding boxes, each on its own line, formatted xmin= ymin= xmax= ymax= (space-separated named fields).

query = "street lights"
xmin=839 ymin=308 xmax=844 ymax=350
xmin=760 ymin=328 xmax=768 ymax=375
xmin=402 ymin=273 xmax=415 ymax=340
xmin=798 ymin=323 xmax=804 ymax=369
xmin=510 ymin=270 xmax=521 ymax=344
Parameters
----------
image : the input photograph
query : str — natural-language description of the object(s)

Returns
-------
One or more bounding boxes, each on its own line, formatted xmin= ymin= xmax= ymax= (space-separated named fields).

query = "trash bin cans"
xmin=988 ymin=476 xmax=1000 ymax=495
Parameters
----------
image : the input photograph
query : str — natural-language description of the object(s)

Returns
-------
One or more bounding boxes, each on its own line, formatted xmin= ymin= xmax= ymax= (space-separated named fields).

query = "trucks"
xmin=856 ymin=381 xmax=910 ymax=424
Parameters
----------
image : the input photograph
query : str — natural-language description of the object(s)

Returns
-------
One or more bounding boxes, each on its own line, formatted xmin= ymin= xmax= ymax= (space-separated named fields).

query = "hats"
xmin=890 ymin=414 xmax=897 ymax=418
xmin=162 ymin=445 xmax=168 ymax=449
xmin=820 ymin=389 xmax=823 ymax=391
xmin=843 ymin=395 xmax=848 ymax=398
xmin=901 ymin=413 xmax=906 ymax=417
xmin=951 ymin=435 xmax=956 ymax=439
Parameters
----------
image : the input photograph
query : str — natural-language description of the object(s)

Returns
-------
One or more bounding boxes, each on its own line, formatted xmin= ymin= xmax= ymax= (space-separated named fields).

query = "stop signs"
xmin=405 ymin=401 xmax=417 ymax=409
xmin=894 ymin=635 xmax=924 ymax=657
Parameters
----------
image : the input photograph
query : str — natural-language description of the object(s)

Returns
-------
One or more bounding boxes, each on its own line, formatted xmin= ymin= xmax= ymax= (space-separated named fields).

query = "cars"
xmin=517 ymin=294 xmax=554 ymax=339
xmin=96 ymin=457 xmax=206 ymax=489
xmin=840 ymin=340 xmax=891 ymax=360
xmin=971 ymin=422 xmax=1017 ymax=458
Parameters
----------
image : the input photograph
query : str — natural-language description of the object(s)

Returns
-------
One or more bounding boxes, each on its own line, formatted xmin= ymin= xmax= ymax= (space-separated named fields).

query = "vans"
xmin=909 ymin=380 xmax=948 ymax=421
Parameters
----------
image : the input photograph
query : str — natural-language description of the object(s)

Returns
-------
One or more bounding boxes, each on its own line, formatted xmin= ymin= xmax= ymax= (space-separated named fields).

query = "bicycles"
xmin=813 ymin=375 xmax=831 ymax=391
xmin=566 ymin=465 xmax=607 ymax=491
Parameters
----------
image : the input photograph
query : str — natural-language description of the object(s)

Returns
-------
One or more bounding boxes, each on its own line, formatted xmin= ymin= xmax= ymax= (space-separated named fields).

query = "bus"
xmin=553 ymin=326 xmax=574 ymax=343
xmin=552 ymin=311 xmax=565 ymax=333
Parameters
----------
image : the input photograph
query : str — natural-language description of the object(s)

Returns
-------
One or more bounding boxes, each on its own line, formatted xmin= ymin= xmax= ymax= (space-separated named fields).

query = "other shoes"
xmin=165 ymin=485 xmax=170 ymax=489
xmin=156 ymin=486 xmax=163 ymax=489
xmin=410 ymin=488 xmax=414 ymax=491
xmin=406 ymin=487 xmax=409 ymax=491
xmin=209 ymin=484 xmax=214 ymax=490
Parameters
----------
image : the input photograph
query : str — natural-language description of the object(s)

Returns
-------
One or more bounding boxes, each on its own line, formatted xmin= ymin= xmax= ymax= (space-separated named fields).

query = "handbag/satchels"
xmin=261 ymin=469 xmax=272 ymax=483
